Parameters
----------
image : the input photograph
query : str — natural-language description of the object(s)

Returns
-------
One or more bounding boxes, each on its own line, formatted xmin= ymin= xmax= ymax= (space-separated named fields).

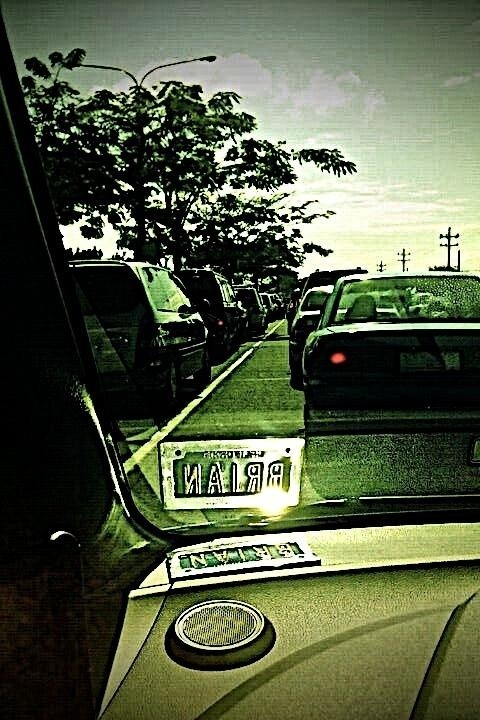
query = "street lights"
xmin=47 ymin=50 xmax=220 ymax=253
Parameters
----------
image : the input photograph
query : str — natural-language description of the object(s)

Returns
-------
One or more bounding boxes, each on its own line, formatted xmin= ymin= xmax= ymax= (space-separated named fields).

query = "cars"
xmin=287 ymin=256 xmax=479 ymax=390
xmin=66 ymin=252 xmax=275 ymax=398
